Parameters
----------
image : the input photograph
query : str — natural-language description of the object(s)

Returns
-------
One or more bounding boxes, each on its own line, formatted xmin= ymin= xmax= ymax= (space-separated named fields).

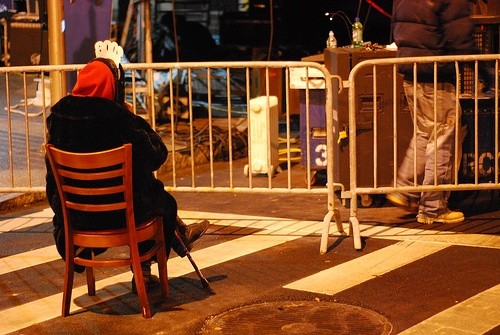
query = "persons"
xmin=160 ymin=13 xmax=216 ymax=61
xmin=386 ymin=0 xmax=499 ymax=224
xmin=45 ymin=58 xmax=211 ymax=296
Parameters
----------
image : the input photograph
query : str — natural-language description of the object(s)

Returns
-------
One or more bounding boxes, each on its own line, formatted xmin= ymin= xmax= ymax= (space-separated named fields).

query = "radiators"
xmin=250 ymin=96 xmax=278 ymax=175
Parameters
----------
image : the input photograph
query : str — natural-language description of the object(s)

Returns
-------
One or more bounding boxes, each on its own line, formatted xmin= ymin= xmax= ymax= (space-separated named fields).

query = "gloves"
xmin=478 ymin=68 xmax=494 ymax=94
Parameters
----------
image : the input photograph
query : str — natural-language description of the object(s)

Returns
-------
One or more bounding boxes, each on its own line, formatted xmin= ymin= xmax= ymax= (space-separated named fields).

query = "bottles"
xmin=352 ymin=17 xmax=363 ymax=48
xmin=326 ymin=31 xmax=337 ymax=48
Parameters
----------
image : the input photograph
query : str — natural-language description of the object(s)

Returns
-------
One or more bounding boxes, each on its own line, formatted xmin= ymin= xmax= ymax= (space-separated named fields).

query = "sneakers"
xmin=416 ymin=207 xmax=465 ymax=225
xmin=386 ymin=191 xmax=419 ymax=212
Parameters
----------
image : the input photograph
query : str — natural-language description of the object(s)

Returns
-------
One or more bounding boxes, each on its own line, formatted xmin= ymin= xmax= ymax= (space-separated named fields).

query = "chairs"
xmin=45 ymin=143 xmax=170 ymax=318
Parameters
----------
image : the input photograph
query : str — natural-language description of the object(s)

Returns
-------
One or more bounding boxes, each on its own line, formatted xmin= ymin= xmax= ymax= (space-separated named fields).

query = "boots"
xmin=171 ymin=215 xmax=210 ymax=258
xmin=130 ymin=263 xmax=159 ymax=295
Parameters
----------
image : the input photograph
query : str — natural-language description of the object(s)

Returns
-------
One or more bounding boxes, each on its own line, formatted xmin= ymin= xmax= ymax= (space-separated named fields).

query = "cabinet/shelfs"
xmin=300 ymin=47 xmax=414 ymax=208
